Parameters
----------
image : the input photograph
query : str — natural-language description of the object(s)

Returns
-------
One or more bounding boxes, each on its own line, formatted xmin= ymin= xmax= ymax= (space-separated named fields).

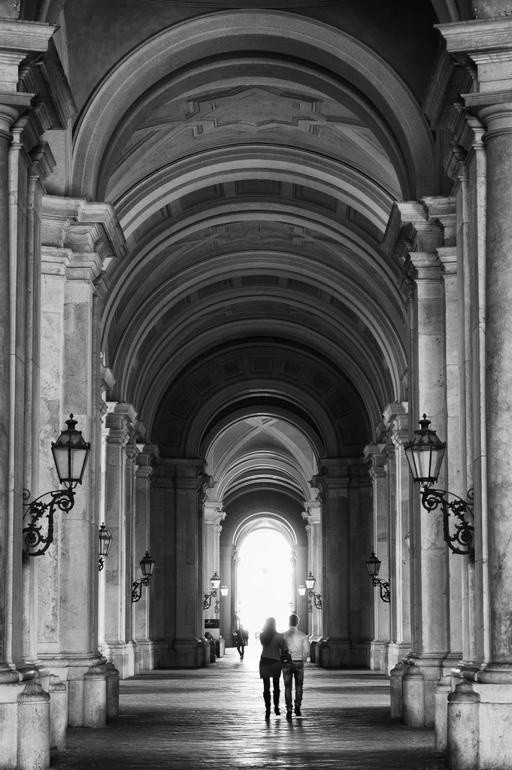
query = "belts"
xmin=292 ymin=660 xmax=303 ymax=662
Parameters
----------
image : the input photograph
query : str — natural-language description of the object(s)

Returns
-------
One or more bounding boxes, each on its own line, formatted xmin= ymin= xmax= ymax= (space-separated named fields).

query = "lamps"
xmin=298 ymin=571 xmax=321 ymax=611
xmin=21 ymin=412 xmax=93 ymax=568
xmin=95 ymin=522 xmax=113 ymax=571
xmin=364 ymin=550 xmax=390 ymax=603
xmin=201 ymin=572 xmax=229 ymax=614
xmin=133 ymin=550 xmax=155 ymax=603
xmin=402 ymin=412 xmax=475 ymax=566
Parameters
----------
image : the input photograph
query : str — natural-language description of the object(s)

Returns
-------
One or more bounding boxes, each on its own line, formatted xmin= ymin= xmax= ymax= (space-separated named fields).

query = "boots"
xmin=263 ymin=691 xmax=270 ymax=717
xmin=273 ymin=689 xmax=280 ymax=715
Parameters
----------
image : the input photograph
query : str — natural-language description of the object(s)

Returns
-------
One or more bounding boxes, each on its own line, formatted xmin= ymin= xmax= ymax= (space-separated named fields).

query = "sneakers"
xmin=287 ymin=709 xmax=292 ymax=717
xmin=295 ymin=709 xmax=301 ymax=716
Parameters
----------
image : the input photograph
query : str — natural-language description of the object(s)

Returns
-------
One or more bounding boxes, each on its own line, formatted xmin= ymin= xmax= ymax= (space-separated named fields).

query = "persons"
xmin=236 ymin=626 xmax=244 ymax=659
xmin=259 ymin=617 xmax=289 ymax=715
xmin=278 ymin=616 xmax=306 ymax=717
